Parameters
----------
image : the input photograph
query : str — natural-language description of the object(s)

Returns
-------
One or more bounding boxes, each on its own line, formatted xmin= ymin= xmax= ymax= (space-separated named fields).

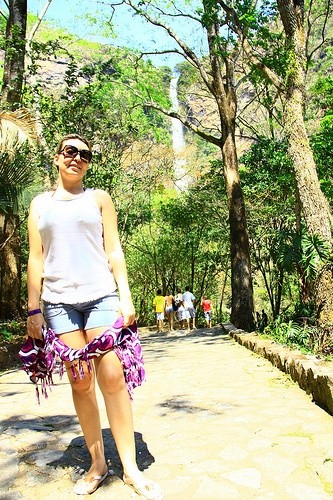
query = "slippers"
xmin=122 ymin=473 xmax=163 ymax=500
xmin=73 ymin=471 xmax=108 ymax=496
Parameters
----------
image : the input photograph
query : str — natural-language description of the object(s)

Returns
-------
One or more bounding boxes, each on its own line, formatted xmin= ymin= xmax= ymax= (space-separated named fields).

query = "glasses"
xmin=58 ymin=144 xmax=92 ymax=163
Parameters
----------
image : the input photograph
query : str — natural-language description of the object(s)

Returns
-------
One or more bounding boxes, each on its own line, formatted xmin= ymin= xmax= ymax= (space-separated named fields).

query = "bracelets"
xmin=27 ymin=308 xmax=42 ymax=317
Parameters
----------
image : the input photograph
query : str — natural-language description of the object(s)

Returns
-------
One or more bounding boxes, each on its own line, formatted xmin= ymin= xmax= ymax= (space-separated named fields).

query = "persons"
xmin=174 ymin=288 xmax=187 ymax=330
xmin=179 ymin=285 xmax=199 ymax=330
xmin=26 ymin=133 xmax=166 ymax=500
xmin=202 ymin=295 xmax=214 ymax=328
xmin=153 ymin=289 xmax=167 ymax=333
xmin=164 ymin=289 xmax=176 ymax=331
